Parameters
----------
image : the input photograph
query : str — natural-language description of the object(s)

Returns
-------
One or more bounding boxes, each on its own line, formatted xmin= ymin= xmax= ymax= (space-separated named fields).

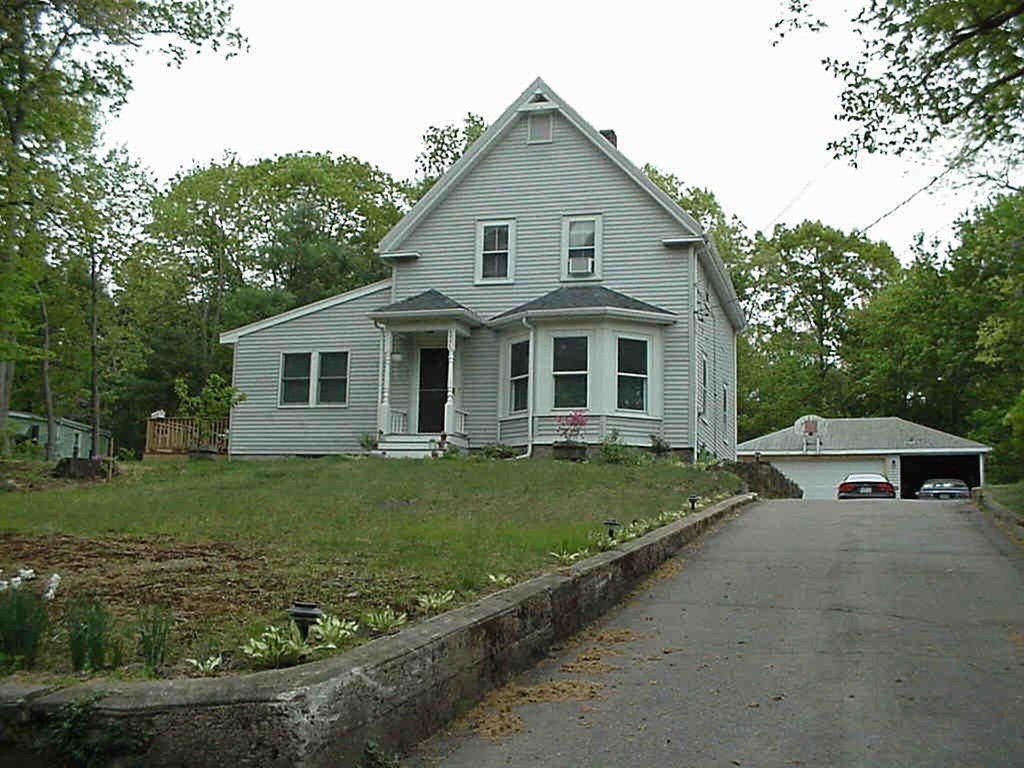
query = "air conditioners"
xmin=570 ymin=256 xmax=593 ymax=274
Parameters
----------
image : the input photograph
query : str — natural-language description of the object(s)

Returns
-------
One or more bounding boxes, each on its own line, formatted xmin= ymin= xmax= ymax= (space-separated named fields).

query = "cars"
xmin=837 ymin=472 xmax=899 ymax=499
xmin=915 ymin=479 xmax=970 ymax=499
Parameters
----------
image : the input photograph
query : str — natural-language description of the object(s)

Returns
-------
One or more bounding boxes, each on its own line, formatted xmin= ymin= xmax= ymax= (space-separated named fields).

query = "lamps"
xmin=390 ymin=335 xmax=404 ymax=363
xmin=603 ymin=519 xmax=619 ymax=538
xmin=284 ymin=601 xmax=323 ymax=642
xmin=688 ymin=495 xmax=699 ymax=512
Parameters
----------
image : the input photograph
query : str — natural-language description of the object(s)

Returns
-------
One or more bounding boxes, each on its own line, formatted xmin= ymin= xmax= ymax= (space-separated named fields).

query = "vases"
xmin=552 ymin=442 xmax=588 ymax=462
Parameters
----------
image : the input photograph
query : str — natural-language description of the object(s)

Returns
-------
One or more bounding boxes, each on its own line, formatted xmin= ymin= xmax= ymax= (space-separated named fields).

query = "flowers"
xmin=555 ymin=410 xmax=589 ymax=444
